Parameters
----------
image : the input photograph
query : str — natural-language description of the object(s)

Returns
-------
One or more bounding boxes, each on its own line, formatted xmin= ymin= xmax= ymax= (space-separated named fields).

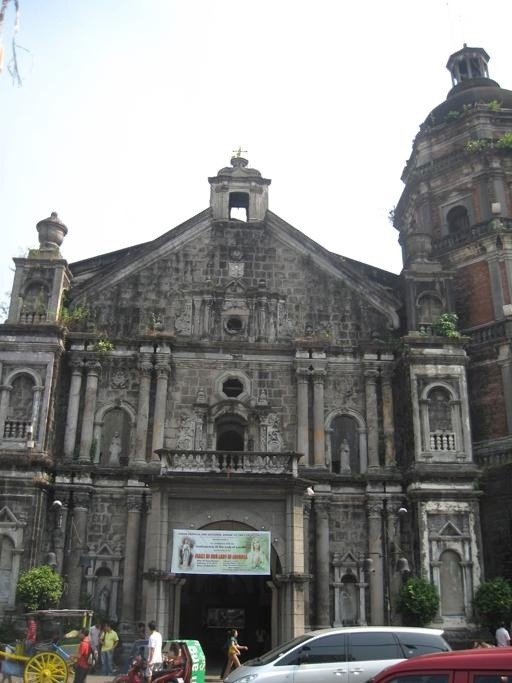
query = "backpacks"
xmin=83 ymin=642 xmax=98 ymax=667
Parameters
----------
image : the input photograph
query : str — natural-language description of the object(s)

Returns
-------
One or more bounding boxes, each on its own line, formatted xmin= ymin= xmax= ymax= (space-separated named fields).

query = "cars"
xmin=366 ymin=647 xmax=512 ymax=683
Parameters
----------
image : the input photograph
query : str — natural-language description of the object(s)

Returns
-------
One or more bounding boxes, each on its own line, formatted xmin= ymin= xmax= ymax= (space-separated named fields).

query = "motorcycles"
xmin=113 ymin=656 xmax=184 ymax=683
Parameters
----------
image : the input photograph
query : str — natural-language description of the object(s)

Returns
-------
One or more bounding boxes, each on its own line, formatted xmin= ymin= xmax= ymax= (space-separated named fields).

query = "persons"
xmin=222 ymin=627 xmax=249 ymax=678
xmin=24 ymin=615 xmax=38 ymax=654
xmin=495 ymin=620 xmax=511 ymax=647
xmin=87 ymin=617 xmax=103 ymax=673
xmin=107 ymin=431 xmax=123 ymax=468
xmin=138 ymin=622 xmax=147 ymax=639
xmin=220 ymin=629 xmax=233 ymax=678
xmin=98 ymin=621 xmax=120 ymax=675
xmin=247 ymin=537 xmax=267 ymax=571
xmin=69 ymin=627 xmax=92 ymax=683
xmin=144 ymin=620 xmax=164 ymax=682
xmin=337 ymin=438 xmax=354 ymax=475
xmin=181 ymin=539 xmax=192 ymax=568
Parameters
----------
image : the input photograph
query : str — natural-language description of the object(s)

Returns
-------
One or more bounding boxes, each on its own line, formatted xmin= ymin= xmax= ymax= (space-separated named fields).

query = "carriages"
xmin=0 ymin=609 xmax=94 ymax=683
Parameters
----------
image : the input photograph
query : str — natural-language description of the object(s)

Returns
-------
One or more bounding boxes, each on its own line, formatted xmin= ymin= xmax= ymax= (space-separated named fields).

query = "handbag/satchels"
xmin=228 ymin=645 xmax=237 ymax=655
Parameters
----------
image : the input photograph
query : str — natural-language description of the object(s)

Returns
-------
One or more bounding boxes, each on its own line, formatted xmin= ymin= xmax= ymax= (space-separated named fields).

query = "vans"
xmin=223 ymin=626 xmax=452 ymax=683
